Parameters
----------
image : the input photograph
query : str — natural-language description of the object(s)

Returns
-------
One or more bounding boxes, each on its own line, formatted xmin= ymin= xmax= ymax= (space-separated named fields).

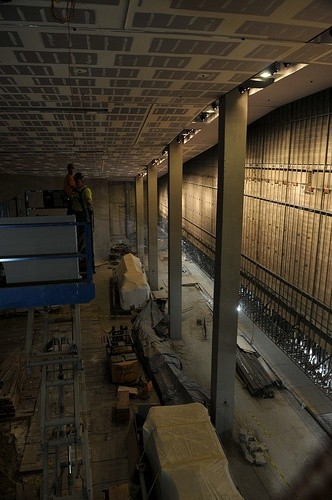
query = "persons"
xmin=63 ymin=164 xmax=76 ymax=209
xmin=71 ymin=173 xmax=93 ymax=213
